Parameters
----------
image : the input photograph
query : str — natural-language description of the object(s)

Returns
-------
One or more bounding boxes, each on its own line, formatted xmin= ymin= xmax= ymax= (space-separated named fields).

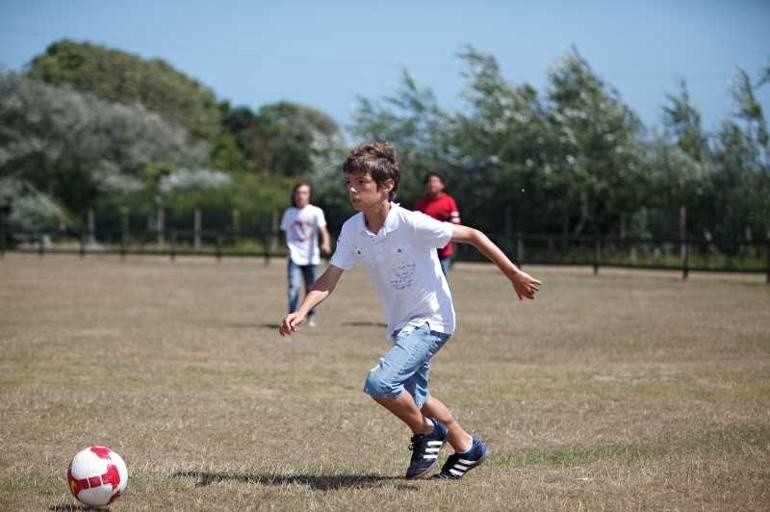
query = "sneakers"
xmin=434 ymin=435 xmax=489 ymax=481
xmin=405 ymin=417 xmax=450 ymax=480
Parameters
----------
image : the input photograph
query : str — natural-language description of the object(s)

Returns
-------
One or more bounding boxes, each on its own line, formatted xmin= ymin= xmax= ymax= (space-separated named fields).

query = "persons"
xmin=408 ymin=168 xmax=462 ymax=271
xmin=271 ymin=136 xmax=544 ymax=481
xmin=274 ymin=179 xmax=334 ymax=330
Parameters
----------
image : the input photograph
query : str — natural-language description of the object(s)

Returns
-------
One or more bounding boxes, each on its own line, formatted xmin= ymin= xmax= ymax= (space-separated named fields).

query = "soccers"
xmin=68 ymin=446 xmax=128 ymax=506
